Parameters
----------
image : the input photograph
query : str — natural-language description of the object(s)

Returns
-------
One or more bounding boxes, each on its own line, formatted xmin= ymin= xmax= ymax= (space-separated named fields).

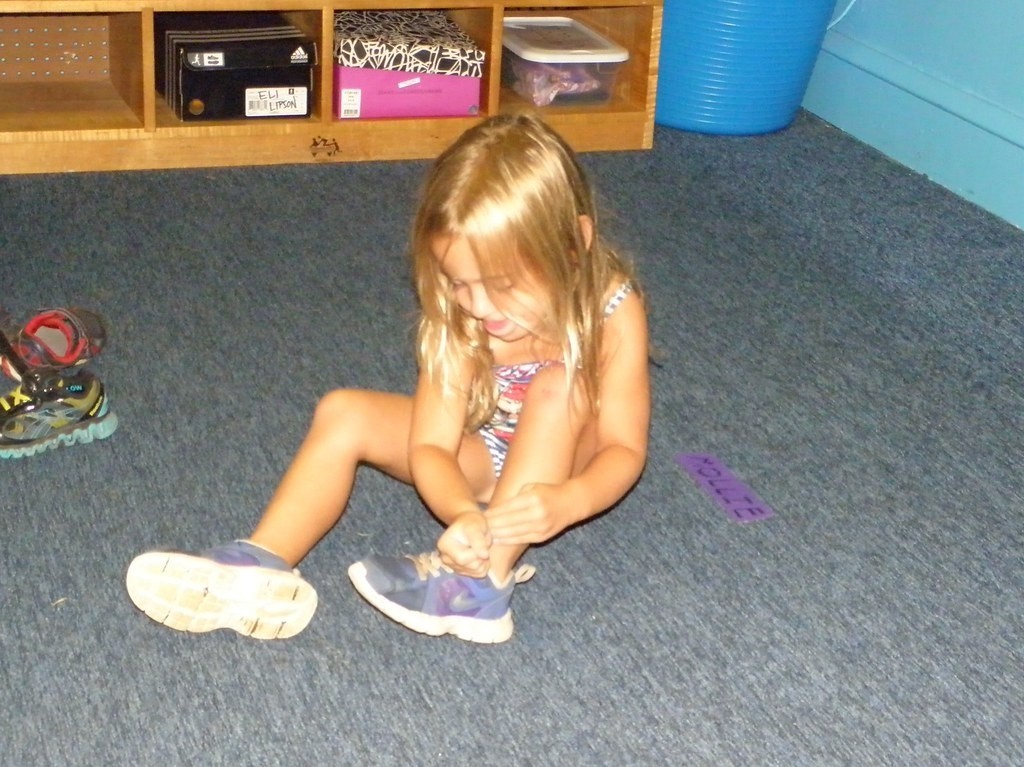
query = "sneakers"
xmin=0 ymin=369 xmax=119 ymax=459
xmin=347 ymin=550 xmax=535 ymax=644
xmin=126 ymin=540 xmax=318 ymax=640
xmin=0 ymin=308 xmax=107 ymax=382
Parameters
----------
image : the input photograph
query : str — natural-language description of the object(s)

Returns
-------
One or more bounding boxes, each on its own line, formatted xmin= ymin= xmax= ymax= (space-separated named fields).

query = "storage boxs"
xmin=502 ymin=16 xmax=630 ymax=107
xmin=334 ymin=10 xmax=487 ymax=120
xmin=154 ymin=11 xmax=319 ymax=122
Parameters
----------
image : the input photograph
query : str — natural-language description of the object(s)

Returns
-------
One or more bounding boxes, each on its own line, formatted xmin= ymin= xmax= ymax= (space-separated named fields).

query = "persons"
xmin=125 ymin=114 xmax=650 ymax=642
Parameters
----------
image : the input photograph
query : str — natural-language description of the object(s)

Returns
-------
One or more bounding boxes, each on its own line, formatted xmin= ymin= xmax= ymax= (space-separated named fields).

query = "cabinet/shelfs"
xmin=0 ymin=0 xmax=662 ymax=175
